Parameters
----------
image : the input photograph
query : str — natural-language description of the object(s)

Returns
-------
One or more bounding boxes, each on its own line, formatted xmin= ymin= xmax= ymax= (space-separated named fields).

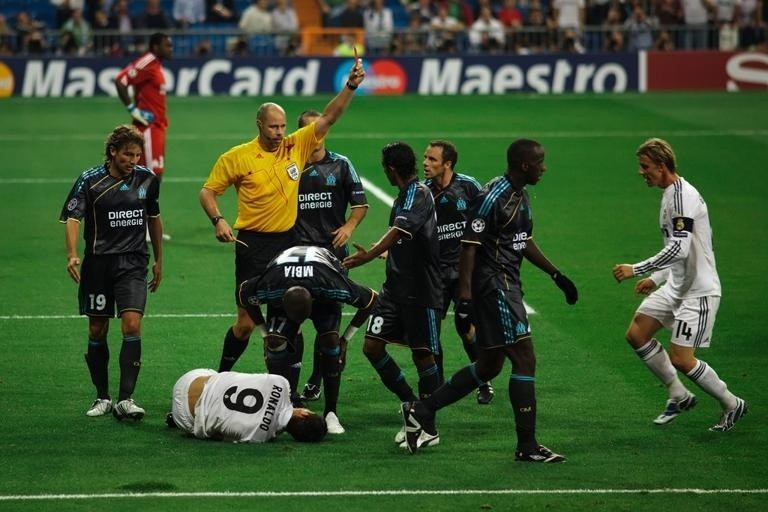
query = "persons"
xmin=339 ymin=137 xmax=443 ymax=448
xmin=319 ymin=0 xmax=768 ymax=57
xmin=396 ymin=137 xmax=581 ymax=463
xmin=111 ymin=30 xmax=174 ymax=242
xmin=238 ymin=243 xmax=380 ymax=434
xmin=286 ymin=105 xmax=370 ymax=403
xmin=198 ymin=57 xmax=369 ymax=375
xmin=163 ymin=366 xmax=331 ymax=445
xmin=0 ymin=0 xmax=301 ymax=59
xmin=607 ymin=135 xmax=750 ymax=435
xmin=412 ymin=141 xmax=497 ymax=406
xmin=57 ymin=124 xmax=165 ymax=421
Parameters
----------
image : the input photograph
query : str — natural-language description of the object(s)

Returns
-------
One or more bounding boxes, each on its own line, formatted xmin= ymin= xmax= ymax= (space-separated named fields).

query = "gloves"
xmin=551 ymin=270 xmax=578 ymax=305
xmin=452 ymin=298 xmax=478 ymax=336
xmin=126 ymin=102 xmax=155 ymax=127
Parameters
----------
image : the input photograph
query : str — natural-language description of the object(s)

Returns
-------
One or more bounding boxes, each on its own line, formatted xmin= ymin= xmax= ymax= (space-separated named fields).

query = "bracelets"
xmin=257 ymin=321 xmax=270 ymax=339
xmin=342 ymin=321 xmax=359 ymax=341
xmin=346 ymin=80 xmax=359 ymax=92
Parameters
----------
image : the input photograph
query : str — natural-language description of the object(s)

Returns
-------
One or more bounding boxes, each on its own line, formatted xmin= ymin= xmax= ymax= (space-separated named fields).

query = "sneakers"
xmin=112 ymin=398 xmax=146 ymax=423
xmin=394 ymin=425 xmax=440 ymax=451
xmin=324 ymin=411 xmax=346 ymax=434
xmin=651 ymin=390 xmax=698 ymax=425
xmin=707 ymin=396 xmax=747 ymax=433
xmin=476 ymin=380 xmax=494 ymax=404
xmin=299 ymin=382 xmax=322 ymax=402
xmin=85 ymin=398 xmax=114 ymax=418
xmin=400 ymin=401 xmax=426 ymax=456
xmin=513 ymin=443 xmax=567 ymax=464
xmin=165 ymin=412 xmax=177 ymax=427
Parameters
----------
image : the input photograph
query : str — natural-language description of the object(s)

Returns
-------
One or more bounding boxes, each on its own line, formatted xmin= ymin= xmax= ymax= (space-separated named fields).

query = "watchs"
xmin=207 ymin=216 xmax=225 ymax=225
